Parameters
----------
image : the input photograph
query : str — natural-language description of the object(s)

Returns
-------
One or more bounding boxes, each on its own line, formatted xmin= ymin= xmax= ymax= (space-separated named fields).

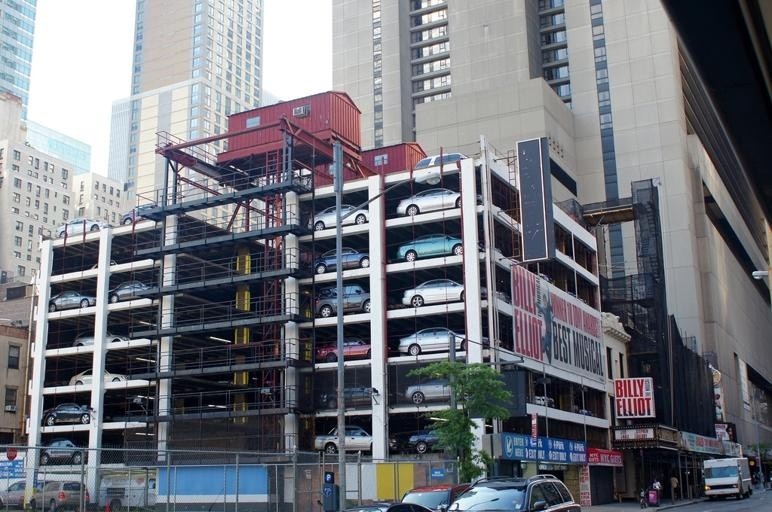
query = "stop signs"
xmin=6 ymin=447 xmax=17 ymax=462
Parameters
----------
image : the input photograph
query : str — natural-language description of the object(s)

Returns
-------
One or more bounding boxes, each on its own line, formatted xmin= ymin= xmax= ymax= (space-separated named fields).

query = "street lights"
xmin=18 ymin=275 xmax=37 ymax=438
xmin=751 ymin=270 xmax=769 ymax=280
xmin=332 ymin=171 xmax=441 ymax=511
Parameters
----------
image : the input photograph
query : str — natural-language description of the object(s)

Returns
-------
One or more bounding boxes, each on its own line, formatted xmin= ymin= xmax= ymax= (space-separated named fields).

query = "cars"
xmin=413 ymin=152 xmax=470 ymax=170
xmin=0 ymin=479 xmax=53 ymax=510
xmin=37 ymin=203 xmax=170 ymax=468
xmin=337 ymin=473 xmax=585 ymax=512
xmin=28 ymin=477 xmax=91 ymax=512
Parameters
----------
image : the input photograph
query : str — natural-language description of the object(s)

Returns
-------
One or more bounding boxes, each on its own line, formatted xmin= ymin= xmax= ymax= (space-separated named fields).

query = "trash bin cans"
xmin=647 ymin=489 xmax=660 ymax=506
xmin=322 ymin=483 xmax=339 ymax=511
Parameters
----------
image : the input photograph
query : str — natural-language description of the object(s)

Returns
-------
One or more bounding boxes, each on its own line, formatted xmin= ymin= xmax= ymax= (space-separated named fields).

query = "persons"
xmin=640 ymin=489 xmax=647 ymax=504
xmin=652 ymin=478 xmax=662 ymax=491
xmin=537 ymin=288 xmax=552 ymax=364
xmin=669 ymin=475 xmax=680 ymax=500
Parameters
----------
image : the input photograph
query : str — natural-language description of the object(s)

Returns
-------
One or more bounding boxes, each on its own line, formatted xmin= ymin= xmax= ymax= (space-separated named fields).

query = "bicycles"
xmin=639 ymin=489 xmax=648 ymax=509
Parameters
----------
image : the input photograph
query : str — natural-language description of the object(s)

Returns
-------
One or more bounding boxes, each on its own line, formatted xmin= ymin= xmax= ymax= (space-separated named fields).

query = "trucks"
xmin=97 ymin=475 xmax=156 ymax=511
xmin=701 ymin=456 xmax=755 ymax=502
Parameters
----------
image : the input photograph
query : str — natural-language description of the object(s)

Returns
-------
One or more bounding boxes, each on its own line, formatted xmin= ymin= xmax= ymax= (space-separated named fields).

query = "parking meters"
xmin=322 ymin=471 xmax=339 ymax=512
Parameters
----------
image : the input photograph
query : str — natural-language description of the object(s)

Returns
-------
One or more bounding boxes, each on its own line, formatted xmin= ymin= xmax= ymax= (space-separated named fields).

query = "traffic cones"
xmin=104 ymin=498 xmax=110 ymax=512
